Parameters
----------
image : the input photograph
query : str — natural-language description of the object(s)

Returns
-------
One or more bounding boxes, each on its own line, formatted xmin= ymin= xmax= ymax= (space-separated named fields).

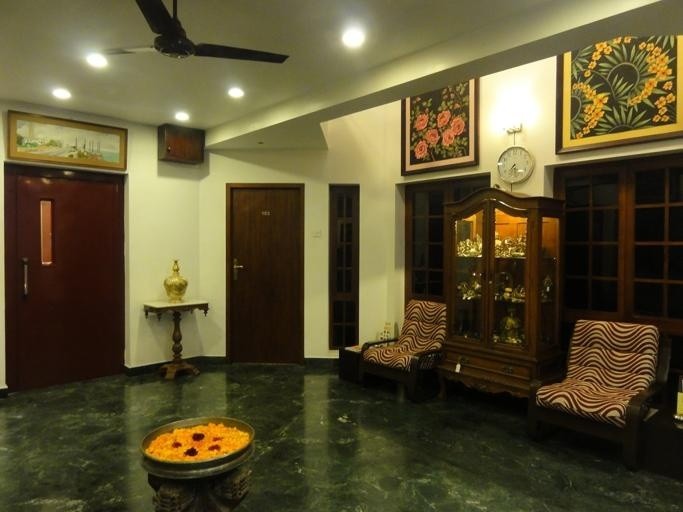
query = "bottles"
xmin=457 ymin=231 xmax=528 ymax=260
xmin=458 ymin=269 xmax=554 ymax=344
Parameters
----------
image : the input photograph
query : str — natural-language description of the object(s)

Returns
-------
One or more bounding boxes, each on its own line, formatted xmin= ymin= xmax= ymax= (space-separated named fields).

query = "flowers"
xmin=410 ymin=81 xmax=469 ymax=162
xmin=572 ymin=37 xmax=677 ymax=139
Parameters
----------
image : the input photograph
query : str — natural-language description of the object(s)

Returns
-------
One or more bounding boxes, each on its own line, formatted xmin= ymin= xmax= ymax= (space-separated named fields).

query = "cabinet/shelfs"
xmin=157 ymin=122 xmax=205 ymax=165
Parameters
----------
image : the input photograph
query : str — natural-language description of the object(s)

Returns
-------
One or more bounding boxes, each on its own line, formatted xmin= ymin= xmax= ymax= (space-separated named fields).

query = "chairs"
xmin=360 ymin=297 xmax=448 ymax=404
xmin=527 ymin=318 xmax=674 ymax=473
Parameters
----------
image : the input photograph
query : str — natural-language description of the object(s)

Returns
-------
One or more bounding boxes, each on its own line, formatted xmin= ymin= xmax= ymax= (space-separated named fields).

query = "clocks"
xmin=496 ymin=146 xmax=535 ymax=185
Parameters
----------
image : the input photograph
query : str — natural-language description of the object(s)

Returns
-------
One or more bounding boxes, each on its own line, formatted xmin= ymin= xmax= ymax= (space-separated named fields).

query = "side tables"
xmin=338 ymin=345 xmax=382 ymax=388
xmin=643 ymin=399 xmax=683 ymax=475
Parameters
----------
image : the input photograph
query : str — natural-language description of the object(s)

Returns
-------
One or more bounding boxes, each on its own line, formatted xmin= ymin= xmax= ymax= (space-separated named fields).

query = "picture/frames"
xmin=401 ymin=76 xmax=480 ymax=177
xmin=555 ymin=31 xmax=683 ymax=155
xmin=6 ymin=108 xmax=128 ymax=171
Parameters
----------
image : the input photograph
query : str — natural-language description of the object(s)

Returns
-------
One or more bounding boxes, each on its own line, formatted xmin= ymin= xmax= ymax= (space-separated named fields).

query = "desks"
xmin=143 ymin=297 xmax=210 ymax=381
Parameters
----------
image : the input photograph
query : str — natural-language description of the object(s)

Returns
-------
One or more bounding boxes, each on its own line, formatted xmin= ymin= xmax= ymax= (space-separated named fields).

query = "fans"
xmin=99 ymin=0 xmax=290 ymax=67
